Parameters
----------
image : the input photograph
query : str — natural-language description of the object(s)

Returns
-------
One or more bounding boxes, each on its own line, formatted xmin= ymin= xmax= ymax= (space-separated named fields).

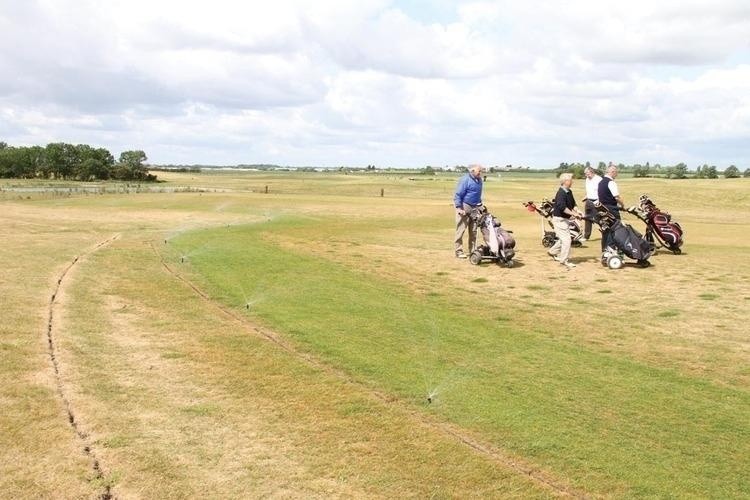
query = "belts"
xmin=589 ymin=198 xmax=597 ymax=201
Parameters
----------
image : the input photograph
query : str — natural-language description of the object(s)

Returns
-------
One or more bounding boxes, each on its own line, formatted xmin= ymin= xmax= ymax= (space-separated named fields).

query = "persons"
xmin=548 ymin=173 xmax=583 ymax=266
xmin=453 ymin=164 xmax=483 ymax=259
xmin=581 ymin=166 xmax=625 ymax=252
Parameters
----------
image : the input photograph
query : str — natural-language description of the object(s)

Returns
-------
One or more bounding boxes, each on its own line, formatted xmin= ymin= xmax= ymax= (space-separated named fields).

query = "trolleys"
xmin=623 ymin=196 xmax=685 ymax=256
xmin=464 ymin=203 xmax=518 ymax=267
xmin=521 ymin=199 xmax=587 ymax=251
xmin=574 ymin=201 xmax=654 ymax=270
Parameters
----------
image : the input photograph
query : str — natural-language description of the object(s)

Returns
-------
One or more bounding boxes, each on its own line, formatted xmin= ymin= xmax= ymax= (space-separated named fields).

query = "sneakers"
xmin=546 ymin=248 xmax=577 ymax=268
xmin=579 ymin=237 xmax=588 ymax=242
xmin=455 ymin=249 xmax=467 ymax=258
